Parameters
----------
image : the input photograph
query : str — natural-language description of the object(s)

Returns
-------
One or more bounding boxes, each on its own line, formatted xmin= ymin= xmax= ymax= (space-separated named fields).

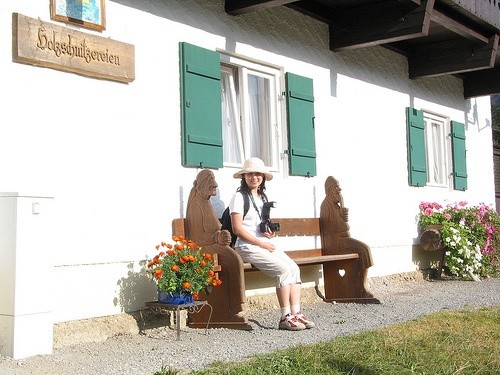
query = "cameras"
xmin=260 ymin=202 xmax=281 ymax=233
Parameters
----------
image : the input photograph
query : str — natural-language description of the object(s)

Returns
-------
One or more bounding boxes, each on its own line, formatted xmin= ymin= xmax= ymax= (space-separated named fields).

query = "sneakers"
xmin=294 ymin=311 xmax=315 ymax=329
xmin=279 ymin=313 xmax=306 ymax=331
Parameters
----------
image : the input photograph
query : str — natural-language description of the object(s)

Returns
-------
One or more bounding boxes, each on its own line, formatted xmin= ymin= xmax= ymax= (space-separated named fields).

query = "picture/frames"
xmin=51 ymin=0 xmax=106 ymax=30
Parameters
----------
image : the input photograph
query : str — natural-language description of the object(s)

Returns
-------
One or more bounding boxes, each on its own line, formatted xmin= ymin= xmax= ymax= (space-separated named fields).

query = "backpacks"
xmin=218 ymin=189 xmax=250 ymax=248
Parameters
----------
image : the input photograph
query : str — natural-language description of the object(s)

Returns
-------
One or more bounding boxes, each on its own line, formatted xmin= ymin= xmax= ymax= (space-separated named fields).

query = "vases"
xmin=157 ymin=291 xmax=193 ymax=304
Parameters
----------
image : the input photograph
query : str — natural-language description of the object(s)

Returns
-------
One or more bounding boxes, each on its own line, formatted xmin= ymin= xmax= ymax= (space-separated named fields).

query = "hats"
xmin=233 ymin=158 xmax=273 ymax=182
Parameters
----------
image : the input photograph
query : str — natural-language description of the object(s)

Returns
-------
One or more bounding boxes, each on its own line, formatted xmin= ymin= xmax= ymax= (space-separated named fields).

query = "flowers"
xmin=146 ymin=237 xmax=222 ymax=299
xmin=418 ymin=200 xmax=500 ymax=282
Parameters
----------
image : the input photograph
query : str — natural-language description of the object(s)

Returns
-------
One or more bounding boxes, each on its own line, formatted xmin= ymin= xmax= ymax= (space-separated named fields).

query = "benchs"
xmin=173 ymin=170 xmax=380 ymax=330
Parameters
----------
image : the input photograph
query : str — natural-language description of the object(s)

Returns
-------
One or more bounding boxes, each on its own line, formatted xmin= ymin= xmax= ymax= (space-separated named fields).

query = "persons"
xmin=229 ymin=157 xmax=315 ymax=330
xmin=186 ymin=170 xmax=245 ymax=311
xmin=319 ymin=176 xmax=374 ymax=292
